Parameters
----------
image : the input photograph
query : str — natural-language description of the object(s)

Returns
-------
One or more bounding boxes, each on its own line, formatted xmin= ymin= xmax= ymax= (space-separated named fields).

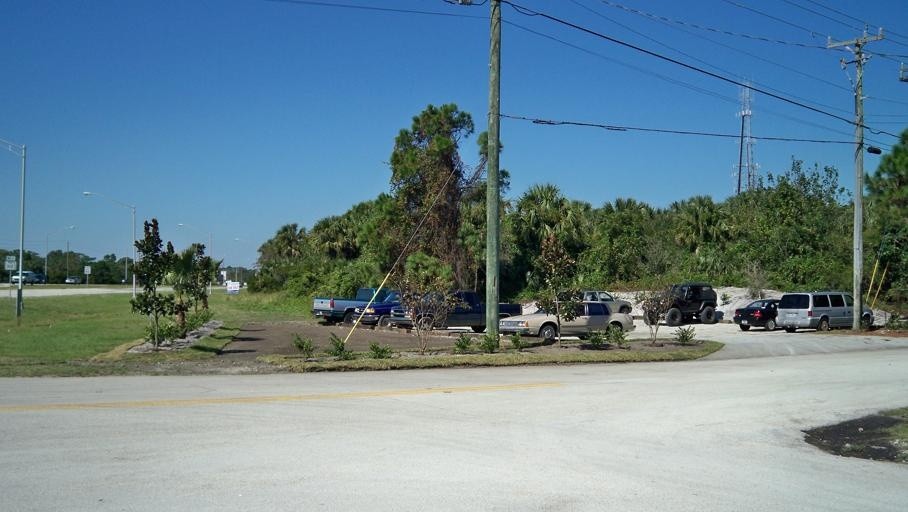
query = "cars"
xmin=64 ymin=275 xmax=82 ymax=284
xmin=11 ymin=271 xmax=46 ymax=285
xmin=499 ymin=290 xmax=636 ymax=339
xmin=733 ymin=299 xmax=780 ymax=332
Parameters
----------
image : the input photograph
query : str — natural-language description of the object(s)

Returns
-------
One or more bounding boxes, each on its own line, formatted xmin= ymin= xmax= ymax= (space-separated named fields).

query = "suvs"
xmin=642 ymin=283 xmax=718 ymax=326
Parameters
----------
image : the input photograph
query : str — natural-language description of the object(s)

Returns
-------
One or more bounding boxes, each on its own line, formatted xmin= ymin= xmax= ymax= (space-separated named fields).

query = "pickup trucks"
xmin=311 ymin=287 xmax=522 ymax=333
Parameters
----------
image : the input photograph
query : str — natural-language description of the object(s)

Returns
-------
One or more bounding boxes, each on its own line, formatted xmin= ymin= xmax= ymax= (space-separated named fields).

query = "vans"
xmin=775 ymin=291 xmax=875 ymax=331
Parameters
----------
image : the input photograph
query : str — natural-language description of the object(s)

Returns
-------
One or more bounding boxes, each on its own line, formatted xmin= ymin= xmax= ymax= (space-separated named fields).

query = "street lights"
xmin=177 ymin=223 xmax=213 ymax=295
xmin=82 ymin=190 xmax=137 ymax=299
